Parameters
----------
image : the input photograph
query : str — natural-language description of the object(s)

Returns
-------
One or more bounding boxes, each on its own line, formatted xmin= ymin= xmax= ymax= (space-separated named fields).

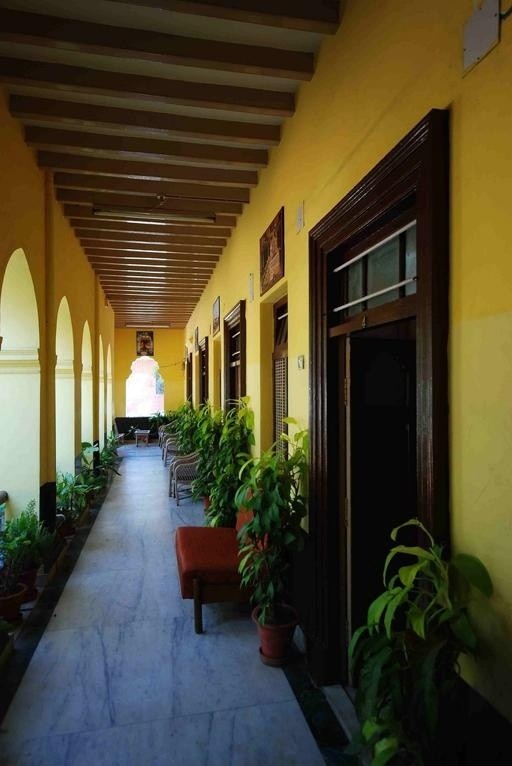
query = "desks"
xmin=134 ymin=429 xmax=149 ymax=449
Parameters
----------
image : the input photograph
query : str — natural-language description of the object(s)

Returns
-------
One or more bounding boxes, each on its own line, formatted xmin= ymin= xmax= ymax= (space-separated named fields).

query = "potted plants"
xmin=191 ymin=394 xmax=257 ymax=529
xmin=230 ymin=417 xmax=312 ymax=663
xmin=0 ymin=430 xmax=125 ymax=659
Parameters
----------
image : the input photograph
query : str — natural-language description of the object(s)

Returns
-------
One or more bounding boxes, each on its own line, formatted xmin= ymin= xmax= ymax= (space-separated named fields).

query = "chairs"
xmin=168 ymin=451 xmax=199 ymax=497
xmin=176 ymin=483 xmax=271 ymax=637
xmin=157 ymin=420 xmax=184 ymax=467
xmin=172 ymin=454 xmax=210 ymax=502
